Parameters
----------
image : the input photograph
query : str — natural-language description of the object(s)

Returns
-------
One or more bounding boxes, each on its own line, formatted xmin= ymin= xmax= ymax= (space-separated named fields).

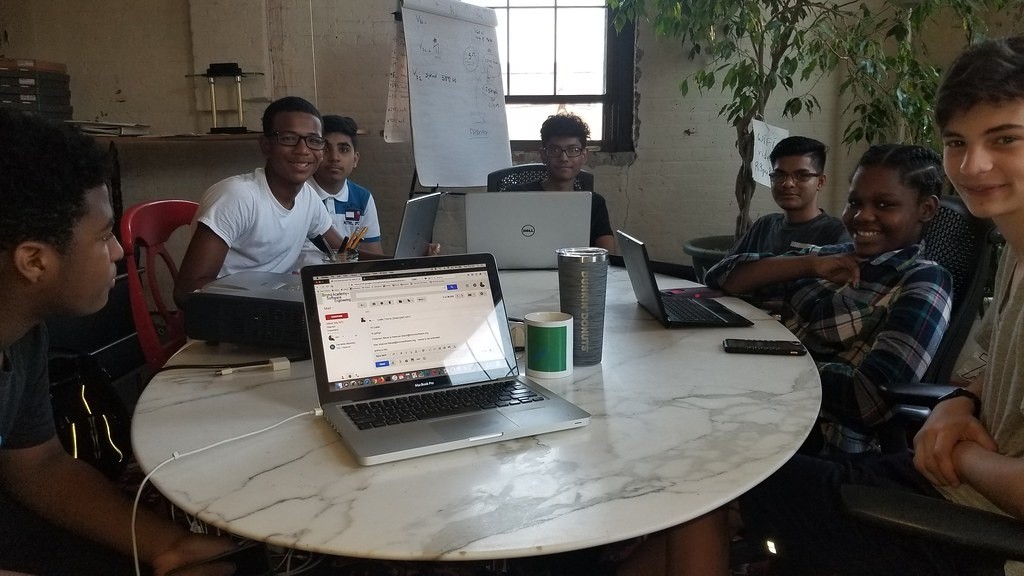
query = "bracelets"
xmin=938 ymin=388 xmax=980 ymax=419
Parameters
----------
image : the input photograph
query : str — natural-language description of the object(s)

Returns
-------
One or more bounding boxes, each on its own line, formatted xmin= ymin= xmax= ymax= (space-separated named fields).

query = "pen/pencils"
xmin=351 ymin=227 xmax=368 ymax=248
xmin=348 ymin=227 xmax=359 ymax=247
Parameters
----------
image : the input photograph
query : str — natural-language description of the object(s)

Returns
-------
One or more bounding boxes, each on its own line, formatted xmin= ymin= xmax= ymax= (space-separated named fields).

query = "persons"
xmin=506 ymin=113 xmax=614 ymax=254
xmin=706 ymin=143 xmax=953 ymax=458
xmin=174 ymin=97 xmax=439 ymax=314
xmin=617 ymin=32 xmax=1023 ymax=576
xmin=724 ymin=137 xmax=851 ymax=320
xmin=0 ymin=103 xmax=240 ymax=576
xmin=294 ymin=115 xmax=381 ymax=273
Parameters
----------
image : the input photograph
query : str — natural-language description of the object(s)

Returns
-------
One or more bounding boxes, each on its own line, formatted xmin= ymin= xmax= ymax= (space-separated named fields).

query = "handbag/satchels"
xmin=44 ymin=348 xmax=132 ymax=479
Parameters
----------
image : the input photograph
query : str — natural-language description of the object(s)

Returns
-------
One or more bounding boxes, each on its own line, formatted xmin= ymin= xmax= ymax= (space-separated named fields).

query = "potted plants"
xmin=610 ymin=0 xmax=1024 ymax=286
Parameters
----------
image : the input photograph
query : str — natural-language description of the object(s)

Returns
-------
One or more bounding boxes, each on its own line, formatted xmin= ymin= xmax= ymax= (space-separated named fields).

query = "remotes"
xmin=723 ymin=339 xmax=807 ymax=355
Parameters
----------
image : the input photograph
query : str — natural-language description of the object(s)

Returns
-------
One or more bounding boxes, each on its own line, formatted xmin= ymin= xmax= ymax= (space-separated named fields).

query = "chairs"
xmin=739 ymin=196 xmax=1023 ymax=576
xmin=118 ymin=199 xmax=202 ymax=371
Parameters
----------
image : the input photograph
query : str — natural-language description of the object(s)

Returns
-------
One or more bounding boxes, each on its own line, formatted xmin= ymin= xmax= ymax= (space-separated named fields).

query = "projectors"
xmin=184 ymin=269 xmax=309 ymax=353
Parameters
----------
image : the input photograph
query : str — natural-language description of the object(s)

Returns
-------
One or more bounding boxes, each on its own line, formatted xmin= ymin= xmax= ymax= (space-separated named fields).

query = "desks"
xmin=131 ymin=264 xmax=822 ymax=575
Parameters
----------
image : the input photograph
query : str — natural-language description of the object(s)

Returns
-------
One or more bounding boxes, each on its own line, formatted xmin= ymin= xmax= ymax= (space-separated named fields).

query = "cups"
xmin=557 ymin=247 xmax=608 ymax=365
xmin=510 ymin=312 xmax=573 ymax=379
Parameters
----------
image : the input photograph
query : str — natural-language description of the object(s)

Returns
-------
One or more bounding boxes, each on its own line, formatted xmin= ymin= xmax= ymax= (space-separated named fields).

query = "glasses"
xmin=268 ymin=130 xmax=328 ymax=151
xmin=543 ymin=144 xmax=583 ymax=157
xmin=769 ymin=171 xmax=821 ymax=183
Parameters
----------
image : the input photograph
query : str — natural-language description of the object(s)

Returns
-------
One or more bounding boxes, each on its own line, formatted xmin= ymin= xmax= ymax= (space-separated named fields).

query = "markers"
xmin=338 ymin=236 xmax=349 ymax=254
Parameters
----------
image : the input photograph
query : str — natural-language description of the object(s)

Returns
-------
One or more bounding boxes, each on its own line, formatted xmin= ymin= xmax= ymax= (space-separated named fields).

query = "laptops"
xmin=301 ymin=251 xmax=593 ymax=466
xmin=466 ymin=191 xmax=592 ymax=271
xmin=616 ymin=229 xmax=755 ymax=329
xmin=394 ymin=192 xmax=441 ymax=258
